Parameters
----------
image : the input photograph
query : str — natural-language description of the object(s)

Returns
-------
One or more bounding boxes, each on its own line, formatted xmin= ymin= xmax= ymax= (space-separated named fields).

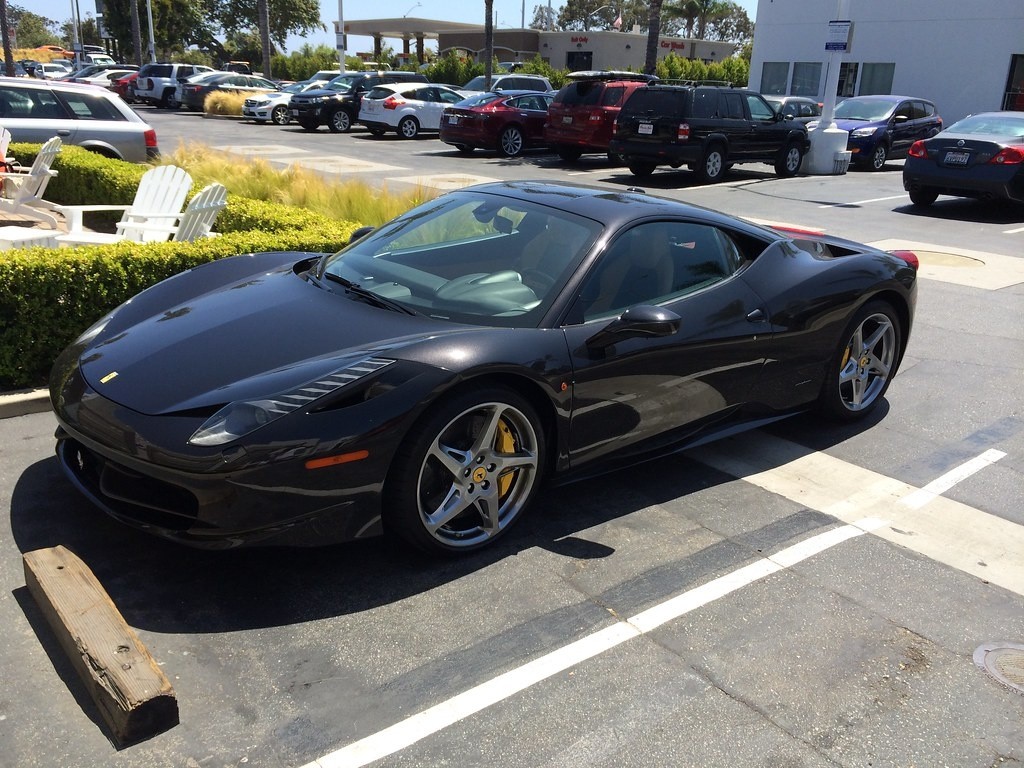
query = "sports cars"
xmin=48 ymin=179 xmax=919 ymax=555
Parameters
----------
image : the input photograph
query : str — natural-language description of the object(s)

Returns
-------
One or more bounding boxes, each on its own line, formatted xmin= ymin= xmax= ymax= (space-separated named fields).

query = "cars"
xmin=0 ymin=44 xmax=660 ymax=126
xmin=439 ymin=90 xmax=555 ymax=157
xmin=543 ymin=79 xmax=656 ymax=167
xmin=902 ymin=112 xmax=1023 ymax=213
xmin=806 ymin=94 xmax=943 ymax=172
xmin=357 ymin=82 xmax=467 ymax=140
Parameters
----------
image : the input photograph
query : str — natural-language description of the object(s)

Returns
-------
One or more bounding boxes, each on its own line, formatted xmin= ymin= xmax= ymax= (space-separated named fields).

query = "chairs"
xmin=584 ymin=224 xmax=674 ymax=317
xmin=0 ymin=126 xmax=15 ymax=173
xmin=52 ymin=164 xmax=192 ymax=241
xmin=56 ymin=180 xmax=231 ymax=243
xmin=0 ymin=99 xmax=69 ymax=118
xmin=0 ymin=135 xmax=64 ymax=228
xmin=510 ymin=215 xmax=590 ymax=303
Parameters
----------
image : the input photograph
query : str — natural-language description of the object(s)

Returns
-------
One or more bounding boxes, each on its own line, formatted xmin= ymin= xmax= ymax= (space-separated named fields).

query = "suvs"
xmin=0 ymin=77 xmax=163 ymax=164
xmin=747 ymin=94 xmax=824 ymax=142
xmin=609 ymin=80 xmax=809 ymax=184
xmin=460 ymin=73 xmax=553 ymax=97
xmin=286 ymin=71 xmax=430 ymax=133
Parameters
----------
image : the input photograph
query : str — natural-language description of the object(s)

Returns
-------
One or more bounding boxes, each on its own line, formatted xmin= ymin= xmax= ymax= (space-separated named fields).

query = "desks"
xmin=0 ymin=225 xmax=64 ymax=252
